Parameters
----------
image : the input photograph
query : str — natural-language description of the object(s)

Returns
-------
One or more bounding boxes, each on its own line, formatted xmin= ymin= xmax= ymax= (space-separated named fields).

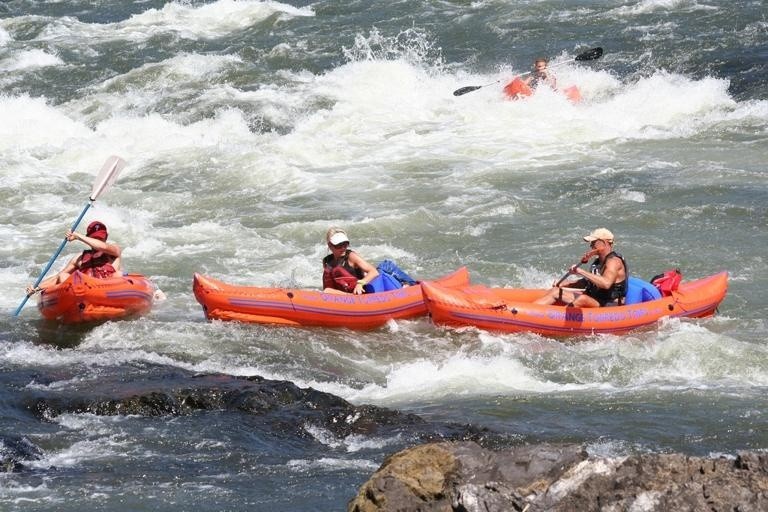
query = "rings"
xmin=359 ymin=290 xmax=361 ymax=292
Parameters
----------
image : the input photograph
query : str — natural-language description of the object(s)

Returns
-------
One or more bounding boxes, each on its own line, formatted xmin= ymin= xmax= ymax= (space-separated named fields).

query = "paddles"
xmin=556 ymin=247 xmax=605 ymax=284
xmin=453 ymin=47 xmax=602 ymax=96
xmin=14 ymin=155 xmax=127 ymax=316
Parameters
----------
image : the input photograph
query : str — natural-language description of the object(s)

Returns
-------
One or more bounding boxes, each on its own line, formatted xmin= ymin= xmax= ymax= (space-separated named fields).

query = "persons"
xmin=321 ymin=226 xmax=380 ymax=295
xmin=523 ymin=57 xmax=555 ymax=94
xmin=25 ymin=220 xmax=122 ymax=298
xmin=532 ymin=227 xmax=628 ymax=308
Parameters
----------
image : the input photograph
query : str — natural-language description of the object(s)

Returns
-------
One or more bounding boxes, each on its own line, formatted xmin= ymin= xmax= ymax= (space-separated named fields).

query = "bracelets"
xmin=356 ymin=279 xmax=367 ymax=286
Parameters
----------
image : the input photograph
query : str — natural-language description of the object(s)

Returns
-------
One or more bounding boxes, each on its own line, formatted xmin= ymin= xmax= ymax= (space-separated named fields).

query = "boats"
xmin=37 ymin=270 xmax=154 ymax=324
xmin=505 ymin=77 xmax=582 ymax=102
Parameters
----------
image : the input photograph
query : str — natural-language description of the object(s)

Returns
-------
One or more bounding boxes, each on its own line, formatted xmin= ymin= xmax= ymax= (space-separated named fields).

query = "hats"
xmin=582 ymin=227 xmax=615 ymax=247
xmin=87 ymin=221 xmax=107 ymax=239
xmin=328 ymin=232 xmax=350 ymax=246
